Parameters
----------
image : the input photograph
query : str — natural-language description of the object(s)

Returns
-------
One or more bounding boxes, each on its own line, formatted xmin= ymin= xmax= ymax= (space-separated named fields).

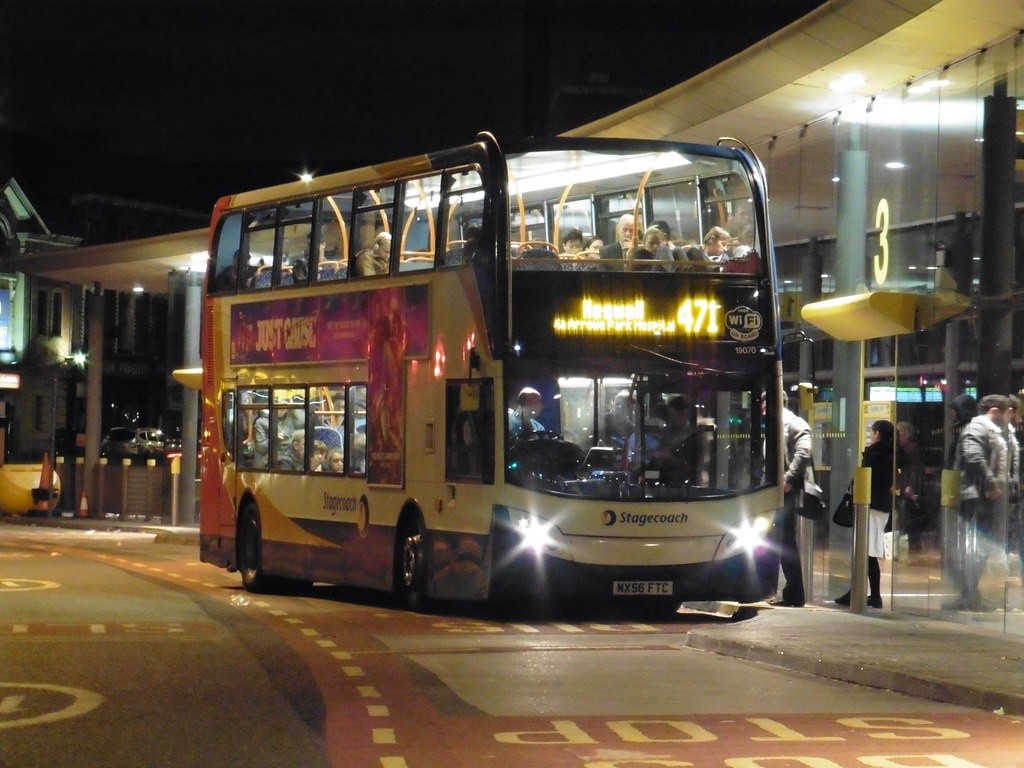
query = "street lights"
xmin=44 ymin=351 xmax=88 ymax=526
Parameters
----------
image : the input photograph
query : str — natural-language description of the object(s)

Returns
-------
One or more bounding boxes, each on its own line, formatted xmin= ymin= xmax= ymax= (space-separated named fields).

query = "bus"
xmin=198 ymin=131 xmax=787 ymax=620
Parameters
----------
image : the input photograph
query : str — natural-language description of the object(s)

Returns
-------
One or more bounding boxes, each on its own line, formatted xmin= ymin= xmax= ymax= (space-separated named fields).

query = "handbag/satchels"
xmin=832 ymin=479 xmax=855 ymax=528
xmin=795 ymin=456 xmax=830 ymax=520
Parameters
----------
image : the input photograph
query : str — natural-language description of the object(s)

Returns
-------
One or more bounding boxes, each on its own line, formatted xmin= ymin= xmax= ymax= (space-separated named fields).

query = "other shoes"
xmin=766 ymin=591 xmax=805 ymax=606
xmin=867 ymin=595 xmax=883 ymax=608
xmin=834 ymin=589 xmax=851 ymax=608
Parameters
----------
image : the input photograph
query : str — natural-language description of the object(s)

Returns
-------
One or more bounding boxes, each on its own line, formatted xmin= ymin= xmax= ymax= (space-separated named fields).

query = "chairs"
xmin=249 ymin=241 xmax=729 ymax=289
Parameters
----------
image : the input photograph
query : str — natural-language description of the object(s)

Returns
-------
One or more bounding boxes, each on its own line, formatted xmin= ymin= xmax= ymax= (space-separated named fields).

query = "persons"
xmin=948 ymin=395 xmax=978 ymax=468
xmin=215 ymin=250 xmax=256 ymax=291
xmin=462 ymin=223 xmax=483 ymax=264
xmin=227 ymin=390 xmax=366 ymax=473
xmin=583 ymin=388 xmax=717 ymax=497
xmin=896 ymin=421 xmax=925 ymax=554
xmin=507 ymin=386 xmax=550 ymax=453
xmin=293 ymin=233 xmax=335 ymax=283
xmin=836 ymin=420 xmax=909 ymax=608
xmin=354 ymin=232 xmax=392 ymax=277
xmin=559 ymin=213 xmax=731 ymax=272
xmin=758 ymin=390 xmax=813 ymax=608
xmin=957 ymin=396 xmax=1020 ymax=612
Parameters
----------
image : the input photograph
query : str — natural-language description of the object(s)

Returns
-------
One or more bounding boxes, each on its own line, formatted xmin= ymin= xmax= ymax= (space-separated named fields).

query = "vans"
xmin=102 ymin=426 xmax=166 ymax=455
